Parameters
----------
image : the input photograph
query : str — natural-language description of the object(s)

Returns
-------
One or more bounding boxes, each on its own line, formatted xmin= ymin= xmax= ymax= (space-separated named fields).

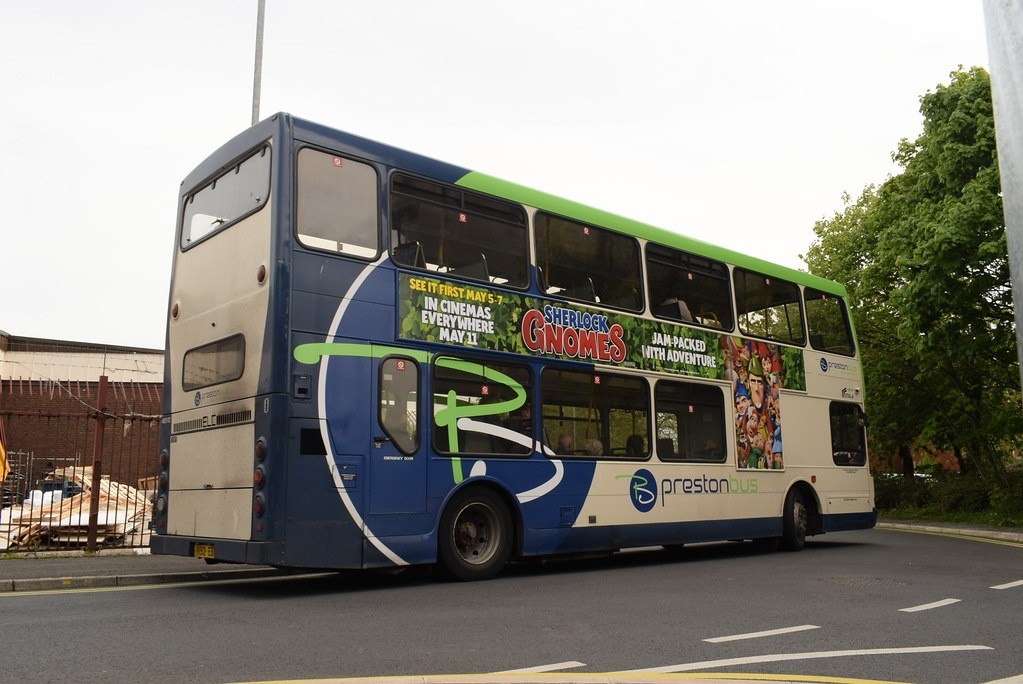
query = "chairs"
xmin=391 ymin=240 xmax=715 ymax=326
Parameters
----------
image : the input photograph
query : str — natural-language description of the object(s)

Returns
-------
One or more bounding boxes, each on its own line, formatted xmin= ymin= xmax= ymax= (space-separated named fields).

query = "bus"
xmin=147 ymin=112 xmax=877 ymax=582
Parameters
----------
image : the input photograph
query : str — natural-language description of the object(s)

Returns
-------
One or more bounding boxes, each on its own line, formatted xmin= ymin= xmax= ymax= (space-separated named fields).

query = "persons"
xmin=555 ymin=430 xmax=572 ymax=455
xmin=506 ymin=404 xmax=530 ymax=444
xmin=649 ymin=288 xmax=700 ymax=323
xmin=582 ymin=438 xmax=608 ymax=457
